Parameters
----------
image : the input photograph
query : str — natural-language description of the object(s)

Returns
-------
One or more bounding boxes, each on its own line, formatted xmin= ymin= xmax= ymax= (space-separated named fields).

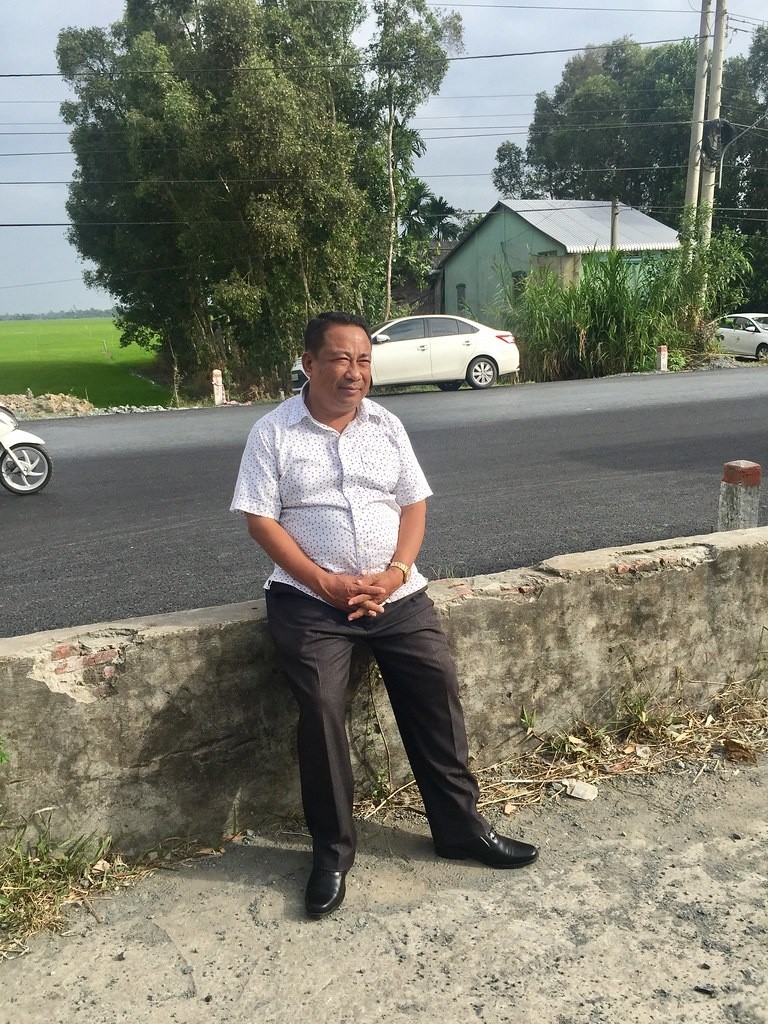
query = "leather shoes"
xmin=305 ymin=864 xmax=347 ymax=921
xmin=435 ymin=827 xmax=539 ymax=869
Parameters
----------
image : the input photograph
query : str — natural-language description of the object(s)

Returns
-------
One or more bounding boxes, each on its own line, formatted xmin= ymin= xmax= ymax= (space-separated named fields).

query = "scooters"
xmin=0 ymin=402 xmax=54 ymax=495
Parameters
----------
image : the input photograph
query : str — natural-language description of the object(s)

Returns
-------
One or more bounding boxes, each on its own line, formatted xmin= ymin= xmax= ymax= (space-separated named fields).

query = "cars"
xmin=289 ymin=313 xmax=521 ymax=395
xmin=703 ymin=312 xmax=768 ymax=363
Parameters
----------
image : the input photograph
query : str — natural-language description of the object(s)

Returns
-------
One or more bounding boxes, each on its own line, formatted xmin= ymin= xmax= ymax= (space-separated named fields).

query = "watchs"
xmin=386 ymin=562 xmax=412 ymax=582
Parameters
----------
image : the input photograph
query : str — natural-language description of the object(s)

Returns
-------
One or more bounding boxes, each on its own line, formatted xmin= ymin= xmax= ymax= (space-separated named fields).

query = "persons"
xmin=229 ymin=312 xmax=538 ymax=918
